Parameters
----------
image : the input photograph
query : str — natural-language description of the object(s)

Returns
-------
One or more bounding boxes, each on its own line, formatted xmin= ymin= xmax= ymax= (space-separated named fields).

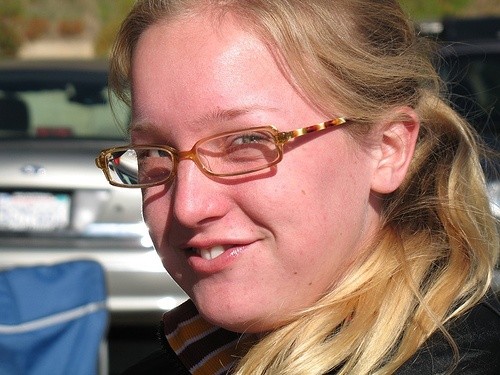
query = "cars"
xmin=0 ymin=57 xmax=190 ymax=332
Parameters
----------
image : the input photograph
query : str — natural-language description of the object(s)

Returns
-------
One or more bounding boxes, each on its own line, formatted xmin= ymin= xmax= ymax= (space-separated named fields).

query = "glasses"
xmin=95 ymin=110 xmax=373 ymax=188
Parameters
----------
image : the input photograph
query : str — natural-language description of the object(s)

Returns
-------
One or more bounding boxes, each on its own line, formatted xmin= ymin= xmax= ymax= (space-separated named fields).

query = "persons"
xmin=95 ymin=0 xmax=500 ymax=375
